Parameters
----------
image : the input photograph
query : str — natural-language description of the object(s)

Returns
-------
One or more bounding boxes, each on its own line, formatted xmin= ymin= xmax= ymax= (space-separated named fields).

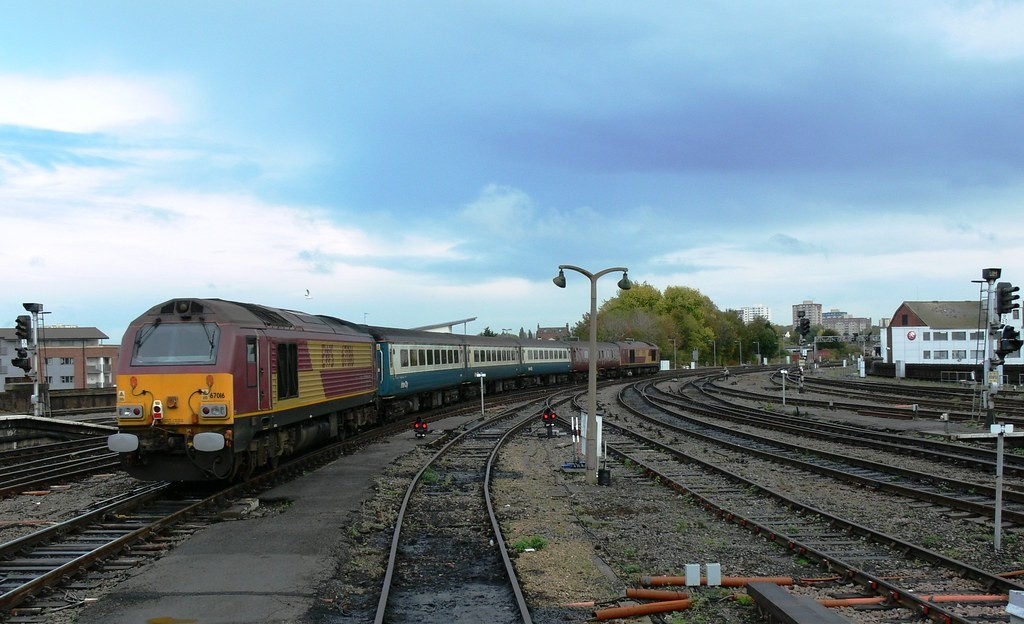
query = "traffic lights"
xmin=996 ymin=282 xmax=1020 ymax=314
xmin=413 ymin=421 xmax=421 ymax=432
xmin=542 ymin=412 xmax=550 ymax=421
xmin=15 ymin=315 xmax=31 ymax=339
xmin=1003 ymin=324 xmax=1020 ymax=339
xmin=550 ymin=412 xmax=558 ymax=421
xmin=22 ymin=303 xmax=38 ymax=313
xmin=420 ymin=421 xmax=429 ymax=431
xmin=800 ymin=318 xmax=810 ymax=335
xmin=11 ymin=358 xmax=31 ymax=372
xmin=15 ymin=347 xmax=27 ymax=358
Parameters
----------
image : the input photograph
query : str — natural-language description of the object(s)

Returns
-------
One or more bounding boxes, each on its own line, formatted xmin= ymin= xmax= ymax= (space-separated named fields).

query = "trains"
xmin=108 ymin=296 xmax=662 ymax=490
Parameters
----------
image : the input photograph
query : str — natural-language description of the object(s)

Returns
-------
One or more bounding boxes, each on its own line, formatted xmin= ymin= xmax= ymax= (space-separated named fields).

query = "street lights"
xmin=982 ymin=268 xmax=1002 ymax=409
xmin=753 ymin=342 xmax=759 ymax=365
xmin=552 ymin=264 xmax=634 ymax=480
xmin=774 ymin=343 xmax=780 ymax=363
xmin=734 ymin=341 xmax=742 ymax=366
xmin=668 ymin=339 xmax=676 ymax=370
xmin=708 ymin=340 xmax=716 ymax=367
